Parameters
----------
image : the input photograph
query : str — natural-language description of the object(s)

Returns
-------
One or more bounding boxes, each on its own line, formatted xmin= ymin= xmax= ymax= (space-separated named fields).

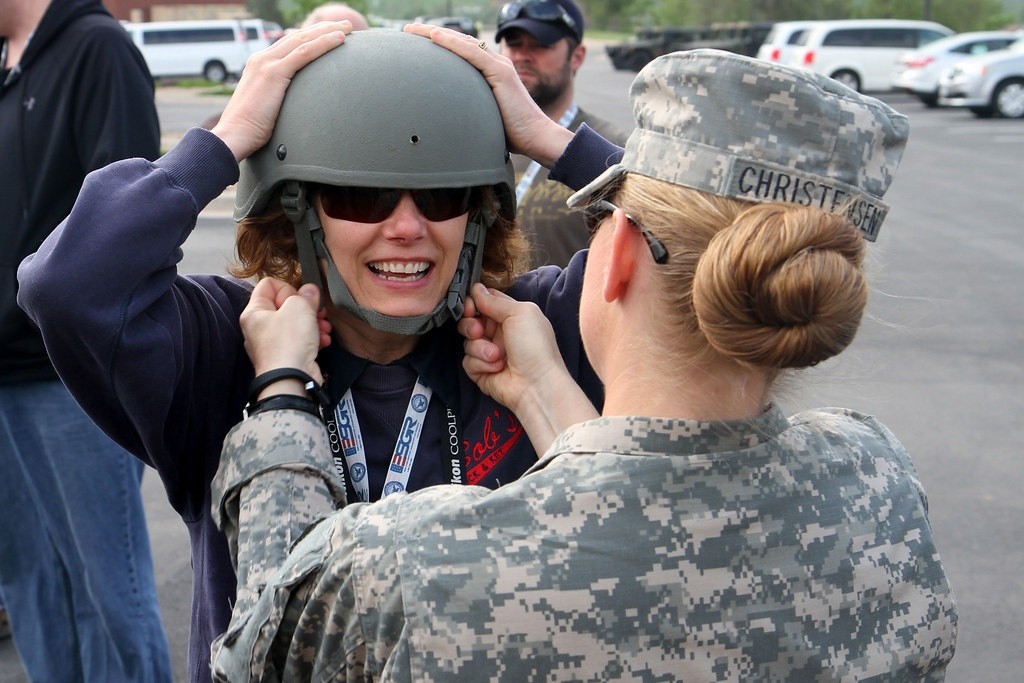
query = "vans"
xmin=789 ymin=18 xmax=957 ymax=93
xmin=122 ymin=18 xmax=280 ymax=83
xmin=758 ymin=21 xmax=812 ymax=74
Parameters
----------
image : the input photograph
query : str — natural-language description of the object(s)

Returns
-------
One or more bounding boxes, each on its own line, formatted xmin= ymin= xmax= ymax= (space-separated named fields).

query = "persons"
xmin=16 ymin=3 xmax=623 ymax=680
xmin=0 ymin=1 xmax=171 ymax=682
xmin=210 ymin=47 xmax=959 ymax=683
xmin=494 ymin=0 xmax=625 ymax=272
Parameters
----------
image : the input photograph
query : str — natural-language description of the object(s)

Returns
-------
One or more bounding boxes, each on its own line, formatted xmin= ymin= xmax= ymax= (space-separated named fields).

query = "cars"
xmin=893 ymin=30 xmax=1024 ymax=103
xmin=940 ymin=42 xmax=1024 ymax=118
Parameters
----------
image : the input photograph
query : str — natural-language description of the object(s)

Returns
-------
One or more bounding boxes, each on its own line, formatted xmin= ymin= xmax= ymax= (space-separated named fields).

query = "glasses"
xmin=496 ymin=0 xmax=582 ymax=45
xmin=299 ymin=179 xmax=475 ymax=222
xmin=584 ymin=174 xmax=668 ymax=264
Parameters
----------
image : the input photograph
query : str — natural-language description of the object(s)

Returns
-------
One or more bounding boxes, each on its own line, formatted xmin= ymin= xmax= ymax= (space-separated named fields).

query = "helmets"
xmin=233 ymin=27 xmax=517 ymax=223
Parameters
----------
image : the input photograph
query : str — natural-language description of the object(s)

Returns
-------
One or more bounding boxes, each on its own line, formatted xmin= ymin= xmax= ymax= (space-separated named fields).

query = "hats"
xmin=494 ymin=0 xmax=584 ymax=48
xmin=565 ymin=49 xmax=909 ymax=243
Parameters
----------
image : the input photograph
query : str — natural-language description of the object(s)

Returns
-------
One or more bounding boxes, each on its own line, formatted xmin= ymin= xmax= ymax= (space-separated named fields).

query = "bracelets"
xmin=243 ymin=368 xmax=336 ymax=426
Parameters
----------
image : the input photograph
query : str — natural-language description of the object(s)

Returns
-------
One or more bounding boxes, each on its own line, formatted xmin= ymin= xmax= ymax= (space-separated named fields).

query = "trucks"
xmin=607 ymin=22 xmax=753 ymax=74
